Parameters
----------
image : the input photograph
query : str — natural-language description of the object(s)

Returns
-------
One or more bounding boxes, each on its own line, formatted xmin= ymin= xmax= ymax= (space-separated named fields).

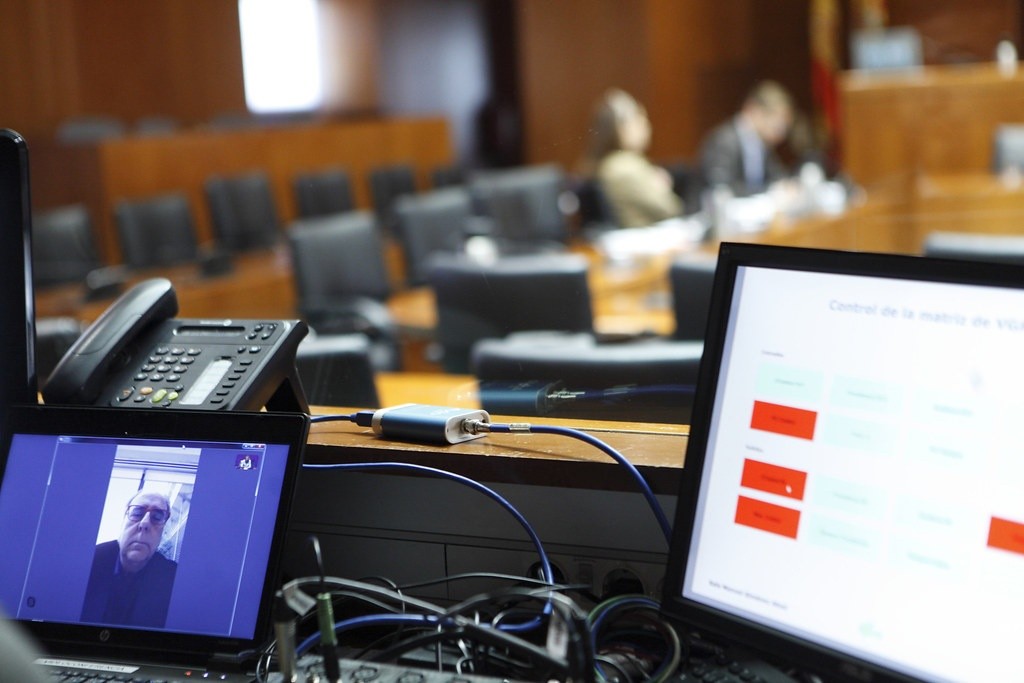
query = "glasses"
xmin=126 ymin=503 xmax=170 ymax=527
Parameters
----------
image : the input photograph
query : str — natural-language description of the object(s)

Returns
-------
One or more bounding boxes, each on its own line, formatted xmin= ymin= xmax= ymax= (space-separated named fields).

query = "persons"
xmin=593 ymin=78 xmax=801 ymax=228
xmin=79 ymin=488 xmax=178 ymax=629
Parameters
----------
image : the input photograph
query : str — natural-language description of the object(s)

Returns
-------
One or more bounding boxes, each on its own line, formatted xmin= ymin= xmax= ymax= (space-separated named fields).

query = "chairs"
xmin=33 ymin=161 xmax=719 ymax=377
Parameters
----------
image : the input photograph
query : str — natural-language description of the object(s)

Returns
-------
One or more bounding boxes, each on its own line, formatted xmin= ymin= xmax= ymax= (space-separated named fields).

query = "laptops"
xmin=0 ymin=403 xmax=309 ymax=683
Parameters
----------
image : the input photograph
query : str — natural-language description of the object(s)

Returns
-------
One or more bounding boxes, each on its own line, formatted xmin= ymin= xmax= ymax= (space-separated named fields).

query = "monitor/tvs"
xmin=663 ymin=243 xmax=1024 ymax=683
